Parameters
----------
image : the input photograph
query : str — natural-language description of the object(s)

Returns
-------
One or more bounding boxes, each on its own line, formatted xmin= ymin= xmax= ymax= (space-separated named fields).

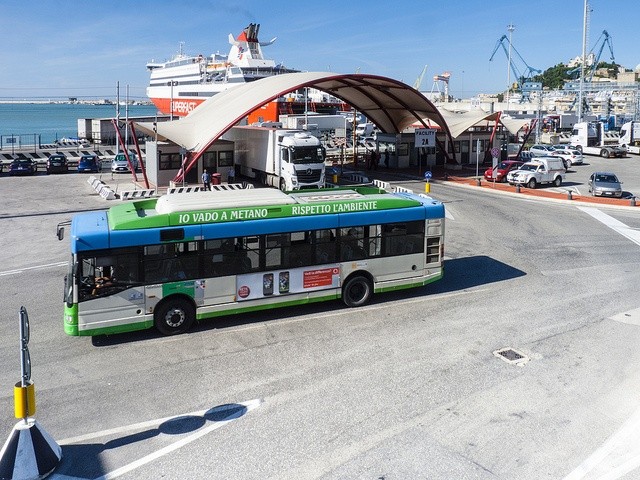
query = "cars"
xmin=79 ymin=156 xmax=101 ymax=172
xmin=530 ymin=144 xmax=555 ymax=158
xmin=548 ymin=149 xmax=583 ymax=166
xmin=588 ymin=171 xmax=623 ymax=197
xmin=11 ymin=157 xmax=37 ymax=175
xmin=484 ymin=160 xmax=524 ymax=182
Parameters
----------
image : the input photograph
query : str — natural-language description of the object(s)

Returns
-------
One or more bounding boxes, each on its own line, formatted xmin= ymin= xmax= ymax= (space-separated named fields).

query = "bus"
xmin=56 ymin=179 xmax=446 ymax=336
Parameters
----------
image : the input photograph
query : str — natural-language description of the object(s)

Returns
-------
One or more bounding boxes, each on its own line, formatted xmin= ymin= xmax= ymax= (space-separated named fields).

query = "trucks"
xmin=543 ymin=115 xmax=596 ymax=131
xmin=222 ymin=126 xmax=326 ymax=192
xmin=620 ymin=120 xmax=640 ymax=155
xmin=507 ymin=157 xmax=567 ymax=188
xmin=355 ymin=123 xmax=375 ymax=136
xmin=570 ymin=122 xmax=628 ymax=158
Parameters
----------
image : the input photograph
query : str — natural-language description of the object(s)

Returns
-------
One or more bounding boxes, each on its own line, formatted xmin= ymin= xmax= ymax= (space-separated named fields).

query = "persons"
xmin=228 ymin=168 xmax=235 ymax=184
xmin=369 ymin=149 xmax=376 ymax=170
xmin=92 ymin=265 xmax=121 ymax=295
xmin=376 ymin=151 xmax=381 ymax=168
xmin=384 ymin=149 xmax=390 ymax=167
xmin=201 ymin=169 xmax=211 ymax=191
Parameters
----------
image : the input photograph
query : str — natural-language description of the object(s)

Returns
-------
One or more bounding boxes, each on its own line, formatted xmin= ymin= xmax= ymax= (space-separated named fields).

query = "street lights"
xmin=506 ymin=23 xmax=516 ymax=118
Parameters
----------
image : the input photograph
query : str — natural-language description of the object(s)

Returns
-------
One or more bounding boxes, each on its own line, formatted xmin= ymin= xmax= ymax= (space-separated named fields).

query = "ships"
xmin=146 ymin=23 xmax=352 ymax=127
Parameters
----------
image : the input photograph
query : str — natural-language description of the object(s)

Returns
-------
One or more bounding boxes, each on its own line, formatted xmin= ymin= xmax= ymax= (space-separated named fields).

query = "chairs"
xmin=143 ymin=245 xmax=200 ymax=282
xmin=382 ymin=227 xmax=423 ymax=253
xmin=291 ymin=234 xmax=337 ymax=265
xmin=204 ymin=241 xmax=250 ymax=274
xmin=340 ymin=229 xmax=366 ymax=258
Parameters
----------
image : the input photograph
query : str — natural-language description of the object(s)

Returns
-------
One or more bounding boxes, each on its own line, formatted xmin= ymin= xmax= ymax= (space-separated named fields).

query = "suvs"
xmin=110 ymin=152 xmax=139 ymax=173
xmin=47 ymin=155 xmax=68 ymax=174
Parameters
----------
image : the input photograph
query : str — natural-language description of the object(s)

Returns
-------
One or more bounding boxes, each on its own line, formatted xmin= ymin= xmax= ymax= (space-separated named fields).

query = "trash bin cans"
xmin=213 ymin=173 xmax=221 ymax=185
